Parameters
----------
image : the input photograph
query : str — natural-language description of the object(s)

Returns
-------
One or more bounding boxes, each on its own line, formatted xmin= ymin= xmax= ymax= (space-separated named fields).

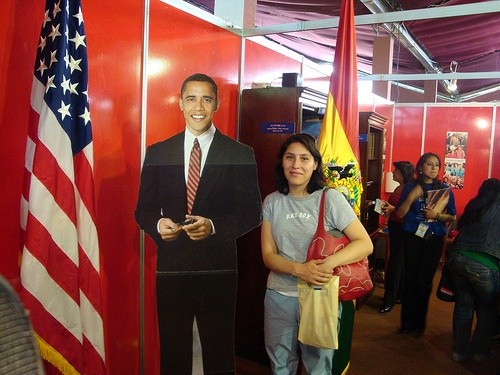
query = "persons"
xmin=450 ymin=198 xmax=500 ymax=366
xmin=444 ymin=131 xmax=468 ymax=190
xmin=259 ymin=132 xmax=374 ymax=375
xmin=133 ymin=72 xmax=264 ymax=375
xmin=377 ymin=160 xmax=418 ymax=315
xmin=392 ymin=152 xmax=457 ymax=337
xmin=435 ymin=177 xmax=500 ymax=343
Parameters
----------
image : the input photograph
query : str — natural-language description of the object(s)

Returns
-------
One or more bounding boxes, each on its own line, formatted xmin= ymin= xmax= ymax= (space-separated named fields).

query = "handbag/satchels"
xmin=297 ymin=274 xmax=340 ymax=350
xmin=311 ymin=187 xmax=374 ymax=300
xmin=436 ymin=265 xmax=455 ymax=302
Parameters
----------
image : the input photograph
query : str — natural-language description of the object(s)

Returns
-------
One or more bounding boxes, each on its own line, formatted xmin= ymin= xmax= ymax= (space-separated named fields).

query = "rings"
xmin=386 ymin=209 xmax=389 ymax=212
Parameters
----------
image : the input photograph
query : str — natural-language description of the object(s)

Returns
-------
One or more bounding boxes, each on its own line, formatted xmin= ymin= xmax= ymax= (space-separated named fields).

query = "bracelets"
xmin=434 ymin=212 xmax=441 ymax=221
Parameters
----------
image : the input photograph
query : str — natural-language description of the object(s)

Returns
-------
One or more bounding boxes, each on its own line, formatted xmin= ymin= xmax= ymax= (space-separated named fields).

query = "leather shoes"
xmin=378 ymin=300 xmax=394 ymax=313
xmin=399 ymin=327 xmax=413 ymax=334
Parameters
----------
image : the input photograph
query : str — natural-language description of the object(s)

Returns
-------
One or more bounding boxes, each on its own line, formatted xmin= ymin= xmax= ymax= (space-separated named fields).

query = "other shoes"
xmin=452 ymin=352 xmax=469 ymax=362
xmin=473 ymin=350 xmax=497 ymax=363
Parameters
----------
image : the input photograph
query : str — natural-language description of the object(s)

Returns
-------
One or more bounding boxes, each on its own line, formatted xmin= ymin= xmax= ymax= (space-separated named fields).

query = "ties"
xmin=186 ymin=138 xmax=201 ymax=215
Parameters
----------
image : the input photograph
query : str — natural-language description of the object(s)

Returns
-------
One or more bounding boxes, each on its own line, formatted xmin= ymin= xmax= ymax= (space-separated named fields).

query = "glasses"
xmin=285 ymin=132 xmax=317 ymax=151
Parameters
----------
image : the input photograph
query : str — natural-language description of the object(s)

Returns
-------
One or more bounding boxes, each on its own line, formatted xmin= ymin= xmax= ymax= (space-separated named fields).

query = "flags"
xmin=16 ymin=0 xmax=113 ymax=375
xmin=315 ymin=0 xmax=365 ymax=374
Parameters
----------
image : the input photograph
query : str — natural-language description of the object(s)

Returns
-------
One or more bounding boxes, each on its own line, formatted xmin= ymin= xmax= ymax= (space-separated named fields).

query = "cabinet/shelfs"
xmin=239 ymin=86 xmax=329 ymax=203
xmin=359 ymin=112 xmax=390 ymax=311
